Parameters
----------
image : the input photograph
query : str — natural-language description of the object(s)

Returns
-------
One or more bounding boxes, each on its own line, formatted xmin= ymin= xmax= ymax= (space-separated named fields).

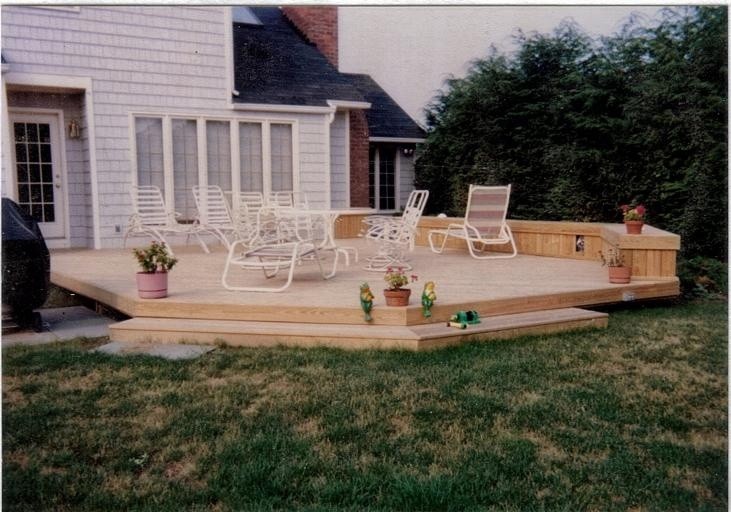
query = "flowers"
xmin=599 ymin=244 xmax=626 ymax=267
xmin=621 ymin=205 xmax=647 ymax=222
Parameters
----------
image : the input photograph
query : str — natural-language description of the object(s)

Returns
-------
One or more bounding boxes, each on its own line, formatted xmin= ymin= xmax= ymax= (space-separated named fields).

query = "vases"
xmin=384 ymin=288 xmax=411 ymax=306
xmin=609 ymin=266 xmax=633 ymax=284
xmin=136 ymin=271 xmax=168 ymax=299
xmin=626 ymin=221 xmax=644 ymax=234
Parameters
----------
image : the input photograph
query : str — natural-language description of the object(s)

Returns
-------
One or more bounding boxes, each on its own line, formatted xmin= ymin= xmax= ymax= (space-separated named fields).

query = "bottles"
xmin=449 ymin=309 xmax=479 ymax=322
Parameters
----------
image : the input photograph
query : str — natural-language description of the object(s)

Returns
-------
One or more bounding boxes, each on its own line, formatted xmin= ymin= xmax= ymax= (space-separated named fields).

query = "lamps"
xmin=69 ymin=120 xmax=81 ymax=139
